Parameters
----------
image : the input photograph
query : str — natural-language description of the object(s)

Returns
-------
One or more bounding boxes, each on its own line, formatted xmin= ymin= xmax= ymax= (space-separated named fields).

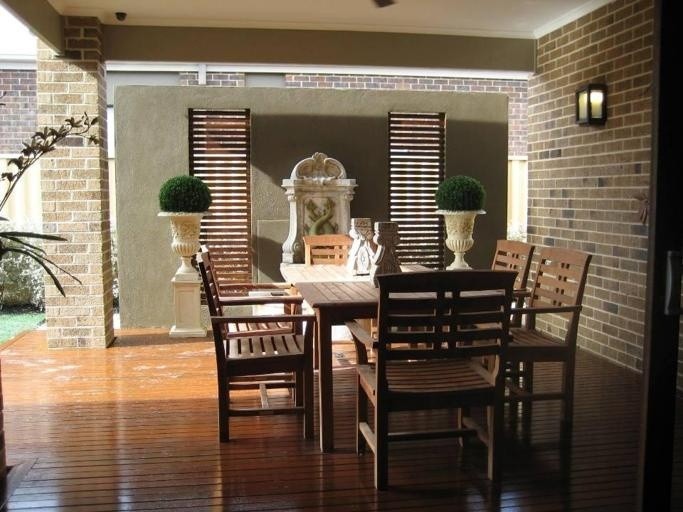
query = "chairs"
xmin=449 ymin=239 xmax=533 ymax=377
xmin=199 ymin=244 xmax=316 ymax=444
xmin=461 ymin=249 xmax=591 ymax=456
xmin=304 ymin=234 xmax=380 ymax=351
xmin=353 ymin=269 xmax=520 ymax=490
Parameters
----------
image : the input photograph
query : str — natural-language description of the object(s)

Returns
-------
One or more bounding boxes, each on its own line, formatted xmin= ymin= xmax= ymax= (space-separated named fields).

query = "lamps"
xmin=575 ymin=83 xmax=607 ymax=126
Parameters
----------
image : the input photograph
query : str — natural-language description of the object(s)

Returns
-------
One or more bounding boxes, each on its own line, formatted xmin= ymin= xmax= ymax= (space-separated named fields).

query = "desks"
xmin=279 ymin=261 xmax=535 ymax=459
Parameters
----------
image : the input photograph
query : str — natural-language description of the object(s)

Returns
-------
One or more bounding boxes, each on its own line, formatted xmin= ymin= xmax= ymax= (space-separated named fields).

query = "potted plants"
xmin=433 ymin=174 xmax=488 ymax=270
xmin=156 ymin=176 xmax=213 ymax=337
